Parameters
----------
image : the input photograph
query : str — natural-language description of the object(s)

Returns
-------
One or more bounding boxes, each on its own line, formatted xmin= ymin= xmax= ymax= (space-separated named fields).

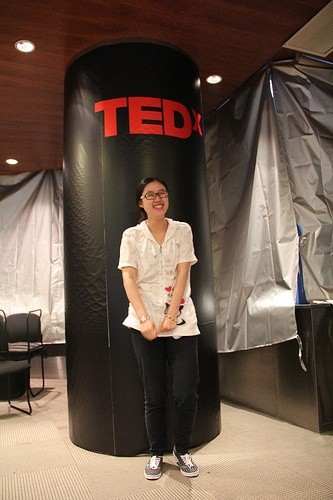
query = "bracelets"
xmin=165 ymin=314 xmax=179 ymax=324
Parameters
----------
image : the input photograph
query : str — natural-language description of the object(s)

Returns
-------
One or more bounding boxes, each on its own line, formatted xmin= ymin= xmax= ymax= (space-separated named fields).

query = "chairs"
xmin=0 ymin=309 xmax=44 ymax=415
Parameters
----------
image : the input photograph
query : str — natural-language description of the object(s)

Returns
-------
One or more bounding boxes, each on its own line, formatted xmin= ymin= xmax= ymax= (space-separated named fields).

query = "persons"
xmin=117 ymin=176 xmax=205 ymax=482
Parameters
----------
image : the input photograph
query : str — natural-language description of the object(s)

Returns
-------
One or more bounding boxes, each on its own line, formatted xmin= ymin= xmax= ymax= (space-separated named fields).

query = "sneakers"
xmin=173 ymin=451 xmax=199 ymax=476
xmin=144 ymin=456 xmax=163 ymax=479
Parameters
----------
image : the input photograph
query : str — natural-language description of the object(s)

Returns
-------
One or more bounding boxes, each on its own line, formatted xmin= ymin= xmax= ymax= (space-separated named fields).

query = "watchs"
xmin=138 ymin=314 xmax=149 ymax=324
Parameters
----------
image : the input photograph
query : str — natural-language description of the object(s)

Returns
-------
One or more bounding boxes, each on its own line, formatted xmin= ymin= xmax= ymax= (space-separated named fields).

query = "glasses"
xmin=141 ymin=190 xmax=168 ymax=200
